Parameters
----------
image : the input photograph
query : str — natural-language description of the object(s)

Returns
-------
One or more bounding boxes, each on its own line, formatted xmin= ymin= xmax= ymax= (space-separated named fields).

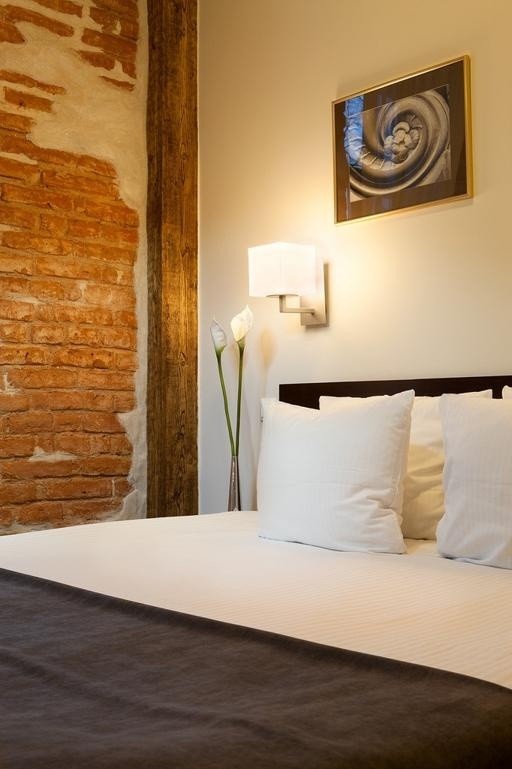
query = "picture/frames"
xmin=331 ymin=55 xmax=474 ymax=224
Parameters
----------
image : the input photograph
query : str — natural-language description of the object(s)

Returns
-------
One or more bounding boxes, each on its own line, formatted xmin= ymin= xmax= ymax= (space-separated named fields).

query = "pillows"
xmin=256 ymin=389 xmax=416 ymax=556
xmin=435 ymin=393 xmax=512 ymax=569
xmin=319 ymin=389 xmax=493 ymax=544
xmin=501 ymin=386 xmax=512 ymax=400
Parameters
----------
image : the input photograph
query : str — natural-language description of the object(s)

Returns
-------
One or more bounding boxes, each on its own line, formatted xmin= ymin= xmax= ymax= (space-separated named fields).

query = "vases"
xmin=228 ymin=456 xmax=241 ymax=511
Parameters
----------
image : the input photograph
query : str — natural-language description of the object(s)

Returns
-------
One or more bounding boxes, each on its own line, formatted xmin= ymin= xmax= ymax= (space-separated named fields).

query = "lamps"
xmin=248 ymin=243 xmax=327 ymax=326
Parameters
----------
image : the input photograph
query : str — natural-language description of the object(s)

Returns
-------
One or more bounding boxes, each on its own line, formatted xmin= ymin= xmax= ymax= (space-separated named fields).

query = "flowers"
xmin=210 ymin=306 xmax=254 ymax=456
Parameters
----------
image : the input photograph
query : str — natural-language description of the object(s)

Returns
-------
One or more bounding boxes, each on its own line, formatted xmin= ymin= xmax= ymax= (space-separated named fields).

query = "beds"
xmin=1 ymin=374 xmax=512 ymax=769
